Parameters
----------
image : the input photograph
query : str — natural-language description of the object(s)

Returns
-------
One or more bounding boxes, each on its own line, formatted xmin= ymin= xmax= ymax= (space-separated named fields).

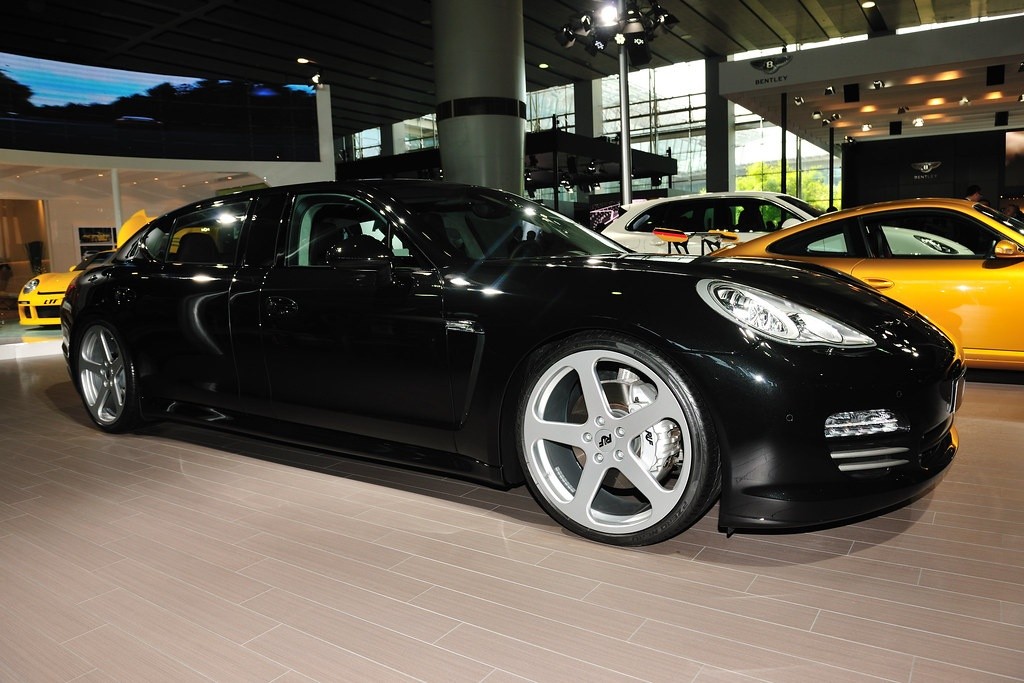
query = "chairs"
xmin=180 ymin=232 xmax=224 ymax=263
xmin=737 ymin=208 xmax=766 ymax=232
xmin=713 ymin=207 xmax=733 ymax=230
xmin=309 ymin=206 xmax=367 ymax=265
xmin=867 ymin=229 xmax=886 ymax=258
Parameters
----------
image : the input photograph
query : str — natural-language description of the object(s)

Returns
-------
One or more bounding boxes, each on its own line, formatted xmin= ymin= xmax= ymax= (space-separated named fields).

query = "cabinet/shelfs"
xmin=74 ymin=225 xmax=119 ymax=271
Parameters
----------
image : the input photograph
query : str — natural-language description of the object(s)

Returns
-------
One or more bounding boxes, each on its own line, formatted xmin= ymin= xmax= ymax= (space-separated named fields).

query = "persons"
xmin=508 ymin=226 xmax=538 ymax=258
xmin=964 ymin=191 xmax=1019 ymax=223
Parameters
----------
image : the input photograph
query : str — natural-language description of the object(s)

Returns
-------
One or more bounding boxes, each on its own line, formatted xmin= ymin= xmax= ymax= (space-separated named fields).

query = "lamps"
xmin=555 ymin=0 xmax=680 ymax=68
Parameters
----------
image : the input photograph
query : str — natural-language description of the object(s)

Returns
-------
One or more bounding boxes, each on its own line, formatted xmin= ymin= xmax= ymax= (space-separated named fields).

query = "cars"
xmin=58 ymin=179 xmax=965 ymax=547
xmin=18 ymin=209 xmax=201 ymax=328
xmin=709 ymin=198 xmax=1024 ymax=370
xmin=600 ymin=190 xmax=973 ymax=258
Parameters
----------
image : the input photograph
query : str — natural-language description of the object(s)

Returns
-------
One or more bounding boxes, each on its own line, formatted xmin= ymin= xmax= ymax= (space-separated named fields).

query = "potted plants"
xmin=0 ymin=265 xmax=13 ymax=291
